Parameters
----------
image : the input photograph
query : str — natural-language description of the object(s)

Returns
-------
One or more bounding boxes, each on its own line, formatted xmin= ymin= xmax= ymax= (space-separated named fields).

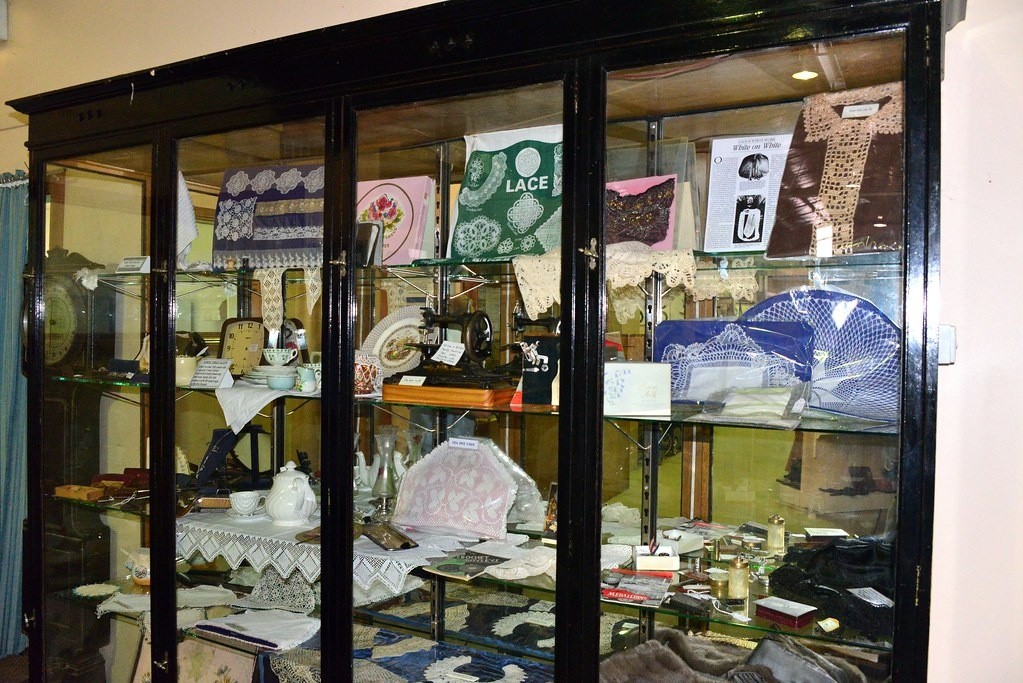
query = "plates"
xmin=226 ymin=507 xmax=267 ymax=519
xmin=242 ymin=364 xmax=297 ymax=385
xmin=359 ymin=307 xmax=440 ymax=379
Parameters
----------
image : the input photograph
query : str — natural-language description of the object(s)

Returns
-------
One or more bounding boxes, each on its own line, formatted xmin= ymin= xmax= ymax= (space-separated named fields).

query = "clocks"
xmin=16 ymin=246 xmax=116 ymax=683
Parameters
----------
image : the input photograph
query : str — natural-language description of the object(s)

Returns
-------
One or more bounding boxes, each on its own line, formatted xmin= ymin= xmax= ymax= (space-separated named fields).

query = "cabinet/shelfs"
xmin=6 ymin=1 xmax=939 ymax=682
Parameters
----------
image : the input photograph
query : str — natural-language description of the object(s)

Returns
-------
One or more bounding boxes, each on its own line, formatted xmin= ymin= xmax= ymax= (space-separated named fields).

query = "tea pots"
xmin=357 ymin=451 xmax=424 ymax=496
xmin=266 ymin=461 xmax=318 ymax=526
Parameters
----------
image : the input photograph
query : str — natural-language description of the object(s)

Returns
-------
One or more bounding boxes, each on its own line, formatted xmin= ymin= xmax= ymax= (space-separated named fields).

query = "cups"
xmin=266 ymin=375 xmax=297 ymax=391
xmin=262 ymin=348 xmax=298 ymax=366
xmin=229 ymin=491 xmax=267 ymax=517
